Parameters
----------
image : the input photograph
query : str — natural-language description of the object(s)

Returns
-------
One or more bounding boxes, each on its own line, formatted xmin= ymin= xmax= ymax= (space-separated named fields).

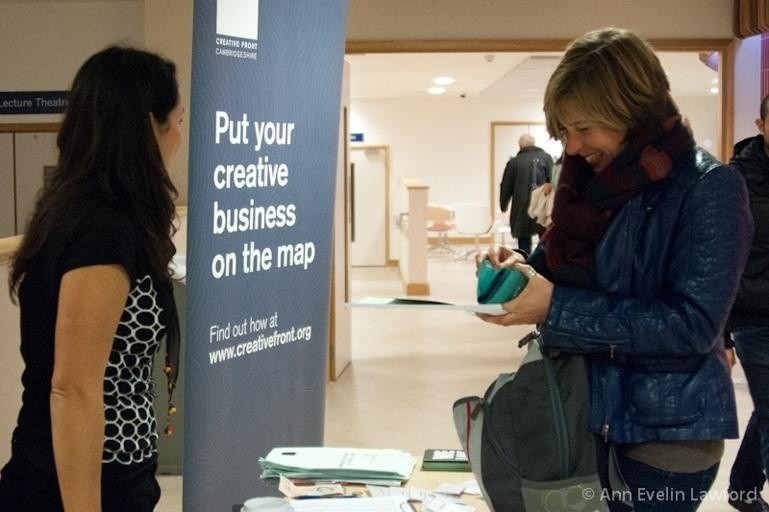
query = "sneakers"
xmin=728 ymin=492 xmax=769 ymax=512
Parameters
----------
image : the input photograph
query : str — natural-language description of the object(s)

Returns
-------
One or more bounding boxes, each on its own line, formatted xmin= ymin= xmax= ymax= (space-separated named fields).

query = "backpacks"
xmin=453 ymin=332 xmax=635 ymax=511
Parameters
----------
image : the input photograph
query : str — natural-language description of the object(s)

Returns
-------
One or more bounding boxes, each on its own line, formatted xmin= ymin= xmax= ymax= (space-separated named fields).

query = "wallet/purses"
xmin=476 ymin=260 xmax=528 ymax=301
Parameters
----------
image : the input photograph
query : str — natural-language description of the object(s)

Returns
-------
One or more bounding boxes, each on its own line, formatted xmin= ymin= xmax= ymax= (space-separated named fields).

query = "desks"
xmin=284 ymin=448 xmax=490 ymax=512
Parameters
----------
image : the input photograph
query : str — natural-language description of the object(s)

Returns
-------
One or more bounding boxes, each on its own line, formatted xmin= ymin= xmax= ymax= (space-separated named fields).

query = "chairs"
xmin=394 ymin=205 xmax=498 ymax=264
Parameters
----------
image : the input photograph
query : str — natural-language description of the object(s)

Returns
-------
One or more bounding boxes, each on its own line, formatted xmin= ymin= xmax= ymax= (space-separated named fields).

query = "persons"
xmin=498 ymin=133 xmax=553 ymax=257
xmin=472 ymin=23 xmax=754 ymax=512
xmin=0 ymin=42 xmax=187 ymax=511
xmin=720 ymin=96 xmax=769 ymax=512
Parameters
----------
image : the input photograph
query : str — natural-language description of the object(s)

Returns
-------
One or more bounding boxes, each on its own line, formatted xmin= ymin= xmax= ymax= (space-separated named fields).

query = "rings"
xmin=524 ymin=268 xmax=538 ymax=279
